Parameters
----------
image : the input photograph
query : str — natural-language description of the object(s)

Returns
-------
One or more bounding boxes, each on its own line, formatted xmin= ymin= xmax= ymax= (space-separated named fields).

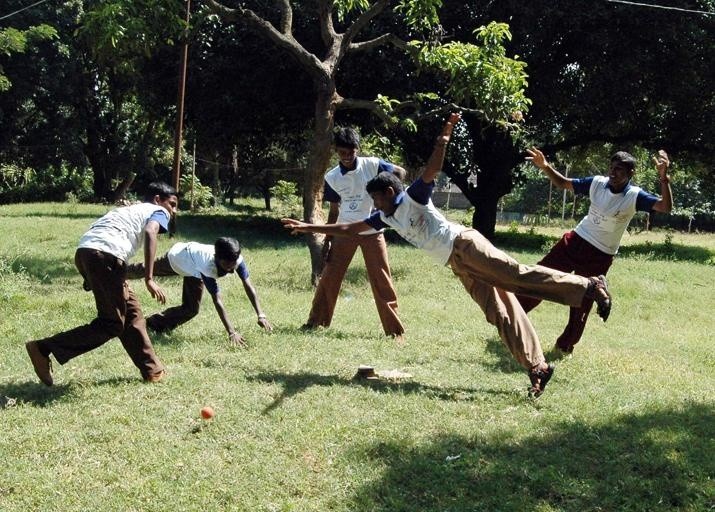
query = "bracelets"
xmin=659 ymin=173 xmax=672 ymax=186
xmin=228 ymin=331 xmax=238 ymax=339
xmin=436 ymin=135 xmax=449 ymax=148
xmin=257 ymin=314 xmax=266 ymax=320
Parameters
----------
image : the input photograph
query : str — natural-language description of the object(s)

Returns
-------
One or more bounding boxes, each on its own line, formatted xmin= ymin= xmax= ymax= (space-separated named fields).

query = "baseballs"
xmin=200 ymin=406 xmax=214 ymax=420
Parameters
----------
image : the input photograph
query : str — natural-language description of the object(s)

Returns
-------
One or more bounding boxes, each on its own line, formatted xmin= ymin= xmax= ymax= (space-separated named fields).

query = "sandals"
xmin=527 ymin=362 xmax=556 ymax=400
xmin=587 ymin=275 xmax=613 ymax=322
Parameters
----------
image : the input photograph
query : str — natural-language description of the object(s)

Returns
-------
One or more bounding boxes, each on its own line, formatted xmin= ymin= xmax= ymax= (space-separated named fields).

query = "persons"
xmin=296 ymin=127 xmax=407 ymax=341
xmin=487 ymin=144 xmax=673 ymax=359
xmin=26 ymin=179 xmax=179 ymax=388
xmin=280 ymin=113 xmax=612 ymax=398
xmin=83 ymin=237 xmax=272 ymax=348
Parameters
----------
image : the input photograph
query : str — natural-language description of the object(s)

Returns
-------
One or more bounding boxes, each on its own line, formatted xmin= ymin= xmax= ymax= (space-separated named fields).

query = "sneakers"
xmin=24 ymin=340 xmax=54 ymax=386
xmin=144 ymin=369 xmax=172 ymax=384
